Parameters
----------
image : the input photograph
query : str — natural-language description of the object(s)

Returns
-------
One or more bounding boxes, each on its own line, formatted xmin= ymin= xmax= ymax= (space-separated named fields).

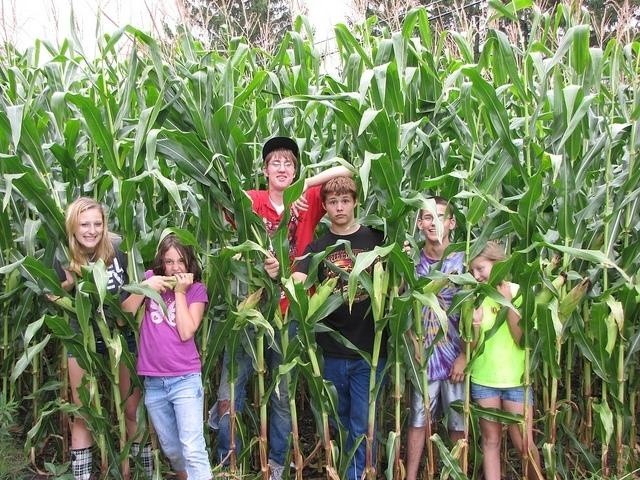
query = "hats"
xmin=263 ymin=136 xmax=298 ymax=160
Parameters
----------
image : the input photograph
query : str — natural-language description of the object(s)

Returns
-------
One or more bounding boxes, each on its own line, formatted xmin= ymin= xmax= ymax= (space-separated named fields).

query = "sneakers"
xmin=268 ymin=458 xmax=285 ymax=480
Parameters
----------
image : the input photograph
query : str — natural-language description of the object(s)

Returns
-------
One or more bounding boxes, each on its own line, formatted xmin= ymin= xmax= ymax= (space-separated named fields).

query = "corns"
xmin=42 ymin=255 xmax=593 ymax=322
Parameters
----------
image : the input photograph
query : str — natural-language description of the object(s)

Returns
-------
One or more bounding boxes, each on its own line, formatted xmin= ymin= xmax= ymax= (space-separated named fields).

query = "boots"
xmin=70 ymin=446 xmax=94 ymax=480
xmin=131 ymin=442 xmax=153 ymax=480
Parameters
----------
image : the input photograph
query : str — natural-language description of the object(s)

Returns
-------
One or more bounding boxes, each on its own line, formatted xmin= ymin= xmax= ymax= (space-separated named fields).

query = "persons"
xmin=402 ymin=198 xmax=467 ymax=480
xmin=116 ymin=232 xmax=213 ymax=480
xmin=460 ymin=242 xmax=540 ymax=480
xmin=53 ymin=197 xmax=155 ymax=480
xmin=206 ymin=136 xmax=353 ymax=480
xmin=265 ymin=176 xmax=388 ymax=480
